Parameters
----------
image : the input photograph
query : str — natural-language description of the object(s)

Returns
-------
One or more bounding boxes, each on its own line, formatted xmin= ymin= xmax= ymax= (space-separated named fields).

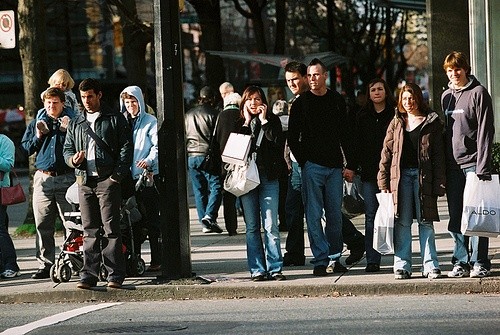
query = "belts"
xmin=39 ymin=169 xmax=72 ymax=176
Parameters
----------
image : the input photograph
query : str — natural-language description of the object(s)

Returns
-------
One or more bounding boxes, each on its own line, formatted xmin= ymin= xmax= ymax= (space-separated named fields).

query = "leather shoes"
xmin=107 ymin=279 xmax=123 ymax=288
xmin=32 ymin=270 xmax=50 ymax=279
xmin=76 ymin=276 xmax=98 ymax=289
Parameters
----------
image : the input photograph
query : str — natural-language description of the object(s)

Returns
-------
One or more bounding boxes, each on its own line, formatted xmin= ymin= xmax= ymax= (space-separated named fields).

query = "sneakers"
xmin=366 ymin=263 xmax=379 ymax=272
xmin=344 ymin=236 xmax=367 ymax=266
xmin=422 ymin=269 xmax=441 ymax=279
xmin=271 ymin=273 xmax=286 ymax=281
xmin=333 ymin=257 xmax=348 ymax=274
xmin=202 ymin=215 xmax=223 ymax=233
xmin=448 ymin=263 xmax=469 ymax=278
xmin=469 ymin=262 xmax=491 ymax=277
xmin=313 ymin=266 xmax=327 ymax=278
xmin=394 ymin=269 xmax=410 ymax=280
xmin=1 ymin=269 xmax=21 ymax=281
xmin=251 ymin=271 xmax=265 ymax=282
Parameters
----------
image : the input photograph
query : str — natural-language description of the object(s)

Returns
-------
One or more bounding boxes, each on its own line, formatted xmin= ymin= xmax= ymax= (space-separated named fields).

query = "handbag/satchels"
xmin=1 ymin=182 xmax=26 ymax=207
xmin=341 ymin=174 xmax=365 ymax=216
xmin=222 ymin=151 xmax=260 ymax=198
xmin=459 ymin=172 xmax=500 ymax=238
xmin=373 ymin=191 xmax=395 ymax=255
xmin=221 ymin=125 xmax=252 ymax=167
xmin=113 ymin=167 xmax=136 ymax=200
xmin=199 ymin=147 xmax=222 ymax=176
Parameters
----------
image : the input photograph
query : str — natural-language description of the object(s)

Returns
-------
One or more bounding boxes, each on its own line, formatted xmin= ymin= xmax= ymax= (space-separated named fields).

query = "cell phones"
xmin=258 ymin=104 xmax=264 ymax=114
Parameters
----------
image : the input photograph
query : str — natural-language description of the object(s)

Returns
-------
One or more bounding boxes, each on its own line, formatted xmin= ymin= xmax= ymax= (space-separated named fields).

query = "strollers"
xmin=50 ymin=197 xmax=145 ymax=283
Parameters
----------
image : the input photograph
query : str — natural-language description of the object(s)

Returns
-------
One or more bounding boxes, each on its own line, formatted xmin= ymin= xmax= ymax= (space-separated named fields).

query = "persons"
xmin=219 ymin=82 xmax=234 ymax=99
xmin=343 ymin=77 xmax=399 ymax=274
xmin=271 ymin=100 xmax=291 ymax=132
xmin=276 ymin=60 xmax=365 ymax=267
xmin=20 ymin=87 xmax=79 ymax=279
xmin=226 ymin=84 xmax=287 ymax=282
xmin=62 ymin=78 xmax=134 ymax=289
xmin=210 ymin=92 xmax=247 ymax=236
xmin=0 ymin=133 xmax=22 ymax=279
xmin=184 ymin=85 xmax=224 ymax=233
xmin=287 ymin=57 xmax=364 ymax=278
xmin=267 ymin=86 xmax=287 ymax=115
xmin=440 ymin=51 xmax=495 ymax=279
xmin=377 ymin=82 xmax=448 ymax=279
xmin=48 ymin=69 xmax=82 ymax=117
xmin=118 ymin=86 xmax=162 ymax=273
